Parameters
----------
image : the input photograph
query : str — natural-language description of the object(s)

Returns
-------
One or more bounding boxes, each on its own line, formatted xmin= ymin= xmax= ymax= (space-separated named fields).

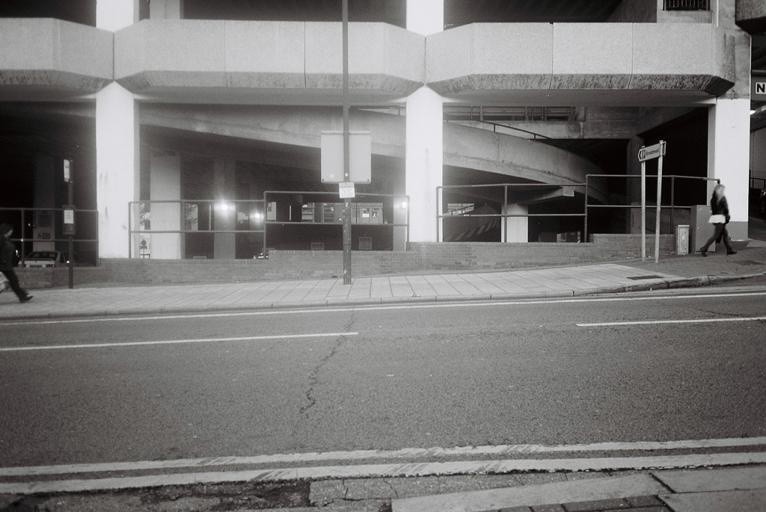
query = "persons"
xmin=700 ymin=184 xmax=737 ymax=257
xmin=0 ymin=221 xmax=34 ymax=303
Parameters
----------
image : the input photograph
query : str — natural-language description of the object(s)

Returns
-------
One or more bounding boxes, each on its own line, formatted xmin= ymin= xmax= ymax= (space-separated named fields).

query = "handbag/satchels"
xmin=708 ymin=213 xmax=726 ymax=224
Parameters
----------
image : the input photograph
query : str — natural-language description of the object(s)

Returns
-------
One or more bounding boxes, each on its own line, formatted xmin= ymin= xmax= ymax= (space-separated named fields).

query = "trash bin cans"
xmin=676 ymin=225 xmax=690 ymax=256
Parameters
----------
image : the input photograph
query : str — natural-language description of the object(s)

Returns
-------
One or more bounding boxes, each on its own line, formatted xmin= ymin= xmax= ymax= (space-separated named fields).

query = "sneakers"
xmin=727 ymin=250 xmax=737 ymax=254
xmin=700 ymin=247 xmax=707 ymax=256
xmin=20 ymin=296 xmax=33 ymax=303
